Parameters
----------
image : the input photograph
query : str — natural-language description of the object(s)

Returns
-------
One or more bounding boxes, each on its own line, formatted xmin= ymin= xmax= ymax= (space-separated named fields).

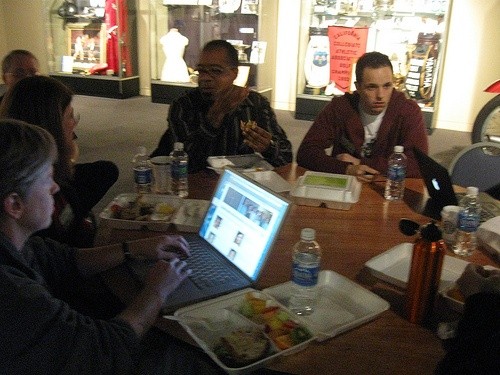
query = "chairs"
xmin=444 ymin=141 xmax=500 ymax=198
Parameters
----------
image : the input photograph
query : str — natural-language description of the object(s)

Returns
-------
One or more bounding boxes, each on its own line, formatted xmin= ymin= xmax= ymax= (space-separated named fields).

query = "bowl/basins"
xmin=364 ymin=242 xmax=500 ymax=314
xmin=99 ymin=192 xmax=210 ymax=233
xmin=206 ymin=152 xmax=292 ymax=193
xmin=477 ymin=216 xmax=500 ymax=257
xmin=290 ymin=170 xmax=361 ymax=210
xmin=174 ymin=270 xmax=390 ymax=374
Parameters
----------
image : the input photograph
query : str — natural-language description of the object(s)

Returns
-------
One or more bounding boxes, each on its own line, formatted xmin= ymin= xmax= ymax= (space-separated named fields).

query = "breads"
xmin=240 ymin=120 xmax=256 ymax=130
xmin=220 ymin=328 xmax=268 ymax=365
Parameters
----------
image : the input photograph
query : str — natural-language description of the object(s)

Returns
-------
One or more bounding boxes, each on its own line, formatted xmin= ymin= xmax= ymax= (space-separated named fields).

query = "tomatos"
xmin=272 ymin=335 xmax=295 ymax=350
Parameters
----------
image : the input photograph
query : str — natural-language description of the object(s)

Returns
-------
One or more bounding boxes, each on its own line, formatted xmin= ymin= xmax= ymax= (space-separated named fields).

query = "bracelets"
xmin=122 ymin=240 xmax=134 ymax=263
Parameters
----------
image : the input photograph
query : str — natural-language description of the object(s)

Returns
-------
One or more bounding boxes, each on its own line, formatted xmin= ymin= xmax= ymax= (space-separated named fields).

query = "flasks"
xmin=397 ymin=218 xmax=448 ymax=325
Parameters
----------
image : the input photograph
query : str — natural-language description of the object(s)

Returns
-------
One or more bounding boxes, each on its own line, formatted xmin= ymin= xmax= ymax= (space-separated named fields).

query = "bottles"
xmin=306 ymin=27 xmax=331 ymax=88
xmin=134 ymin=153 xmax=153 ymax=194
xmin=289 ymin=227 xmax=321 ymax=315
xmin=384 ymin=146 xmax=407 ymax=200
xmin=169 ymin=141 xmax=189 ymax=197
xmin=406 ymin=32 xmax=442 ymax=100
xmin=454 ymin=186 xmax=481 ymax=257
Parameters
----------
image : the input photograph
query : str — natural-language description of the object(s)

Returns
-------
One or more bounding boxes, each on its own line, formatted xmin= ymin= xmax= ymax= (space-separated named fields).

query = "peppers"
xmin=292 ymin=327 xmax=312 ymax=343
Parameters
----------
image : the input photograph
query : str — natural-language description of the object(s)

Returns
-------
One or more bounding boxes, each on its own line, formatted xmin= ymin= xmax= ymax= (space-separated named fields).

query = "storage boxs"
xmin=206 ymin=150 xmax=293 ymax=196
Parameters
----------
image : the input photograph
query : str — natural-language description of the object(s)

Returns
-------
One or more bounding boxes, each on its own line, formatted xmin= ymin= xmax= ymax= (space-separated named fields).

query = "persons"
xmin=160 ymin=27 xmax=190 ymax=81
xmin=0 ymin=75 xmax=76 ymax=303
xmin=295 ymin=52 xmax=428 ymax=183
xmin=1 ymin=50 xmax=119 ymax=242
xmin=152 ymin=40 xmax=292 ymax=167
xmin=0 ymin=118 xmax=193 ymax=375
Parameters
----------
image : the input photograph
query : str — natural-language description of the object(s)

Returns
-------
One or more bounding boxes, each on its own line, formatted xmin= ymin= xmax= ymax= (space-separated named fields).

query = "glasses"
xmin=4 ymin=70 xmax=39 ymax=79
xmin=64 ymin=111 xmax=80 ymax=125
xmin=193 ymin=66 xmax=236 ymax=77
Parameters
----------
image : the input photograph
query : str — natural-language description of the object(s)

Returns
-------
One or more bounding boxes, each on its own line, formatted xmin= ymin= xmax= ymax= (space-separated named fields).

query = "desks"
xmin=90 ymin=152 xmax=500 ymax=375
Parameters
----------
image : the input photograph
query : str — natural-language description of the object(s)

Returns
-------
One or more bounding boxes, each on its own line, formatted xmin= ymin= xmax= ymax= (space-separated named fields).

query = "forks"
xmin=163 ymin=315 xmax=225 ymax=331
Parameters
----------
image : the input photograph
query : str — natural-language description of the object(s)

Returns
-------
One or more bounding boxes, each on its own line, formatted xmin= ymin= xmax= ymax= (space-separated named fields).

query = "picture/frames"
xmin=66 ymin=22 xmax=107 ymax=68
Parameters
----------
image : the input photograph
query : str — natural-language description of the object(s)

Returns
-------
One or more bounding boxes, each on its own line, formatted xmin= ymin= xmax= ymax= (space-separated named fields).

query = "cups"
xmin=328 ymin=25 xmax=368 ymax=95
xmin=441 ymin=205 xmax=464 ymax=243
xmin=151 ymin=156 xmax=169 ymax=193
